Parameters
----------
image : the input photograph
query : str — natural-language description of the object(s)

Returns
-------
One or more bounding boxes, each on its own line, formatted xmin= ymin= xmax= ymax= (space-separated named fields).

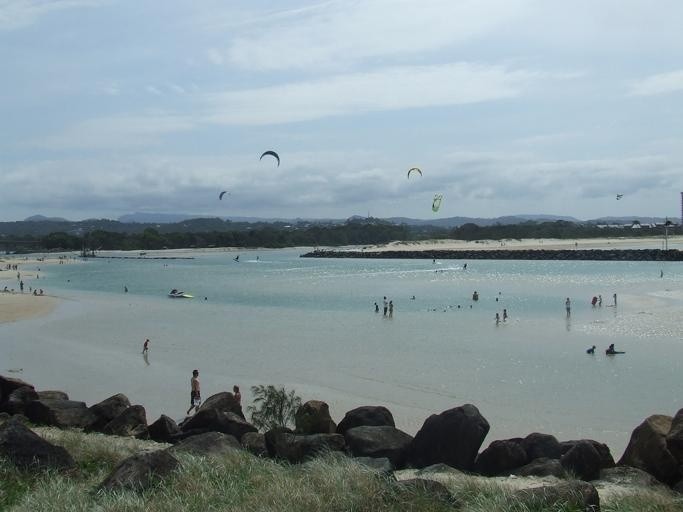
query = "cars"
xmin=139 ymin=250 xmax=145 ymax=256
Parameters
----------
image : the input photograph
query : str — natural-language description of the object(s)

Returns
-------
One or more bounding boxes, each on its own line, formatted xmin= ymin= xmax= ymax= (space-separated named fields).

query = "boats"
xmin=168 ymin=291 xmax=195 ymax=300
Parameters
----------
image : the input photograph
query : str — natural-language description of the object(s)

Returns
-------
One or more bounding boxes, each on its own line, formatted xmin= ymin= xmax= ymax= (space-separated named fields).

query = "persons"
xmin=373 ymin=239 xmax=629 ymax=356
